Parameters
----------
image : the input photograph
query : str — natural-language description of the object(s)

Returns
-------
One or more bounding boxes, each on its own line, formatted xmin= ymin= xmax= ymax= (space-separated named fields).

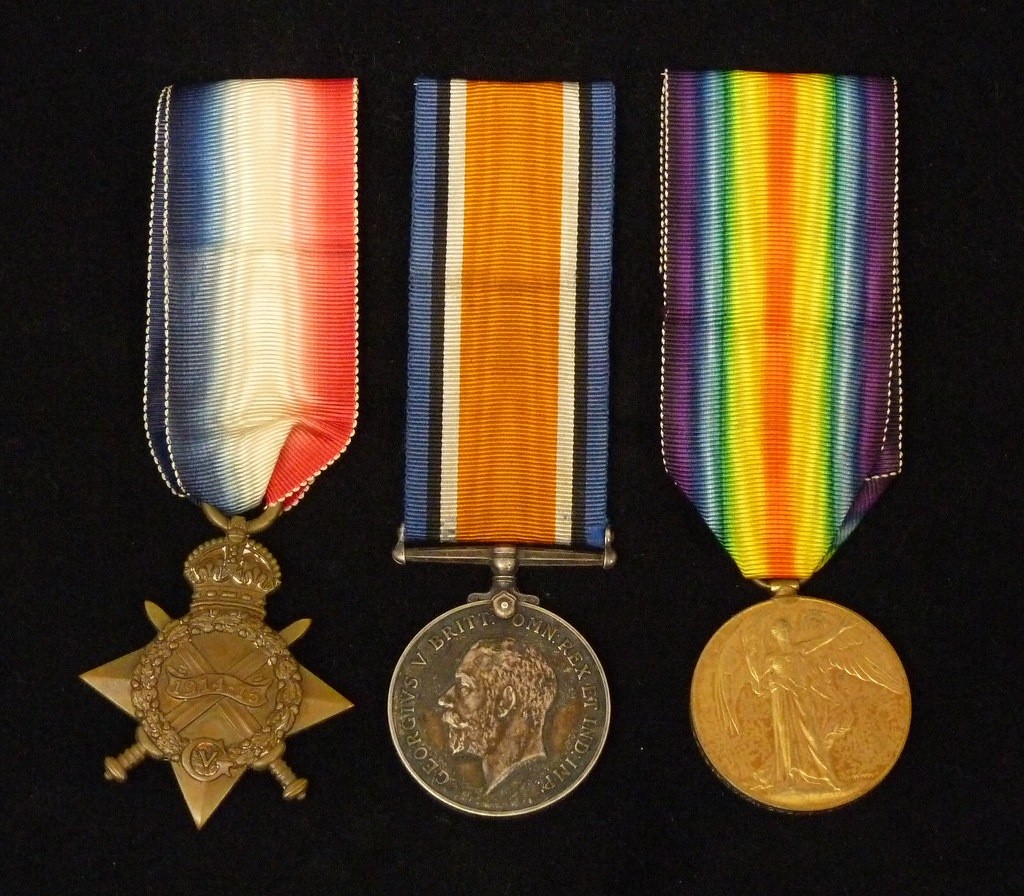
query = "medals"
xmin=386 ymin=599 xmax=613 ymax=817
xmin=690 ymin=597 xmax=913 ymax=816
xmin=79 ymin=536 xmax=353 ymax=830
